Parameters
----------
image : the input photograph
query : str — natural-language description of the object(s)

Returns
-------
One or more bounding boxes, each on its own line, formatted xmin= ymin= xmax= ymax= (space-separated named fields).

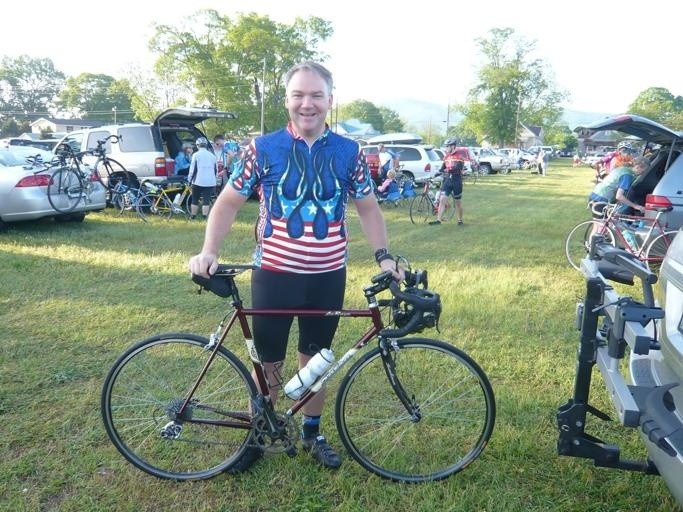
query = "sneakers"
xmin=428 ymin=220 xmax=441 ymax=225
xmin=457 ymin=220 xmax=462 ymax=225
xmin=300 ymin=432 xmax=340 ymax=467
xmin=226 ymin=437 xmax=264 ymax=474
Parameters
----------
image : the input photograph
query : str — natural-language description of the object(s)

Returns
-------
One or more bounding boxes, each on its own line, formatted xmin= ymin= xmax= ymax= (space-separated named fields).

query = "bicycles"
xmin=99 ymin=253 xmax=497 ymax=485
xmin=24 ymin=134 xmax=213 ymax=223
xmin=409 ymin=176 xmax=457 ymax=227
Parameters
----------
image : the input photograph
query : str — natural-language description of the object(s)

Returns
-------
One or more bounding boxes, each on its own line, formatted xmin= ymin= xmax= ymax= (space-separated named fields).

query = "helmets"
xmin=195 ymin=137 xmax=207 ymax=145
xmin=443 ymin=138 xmax=455 ymax=150
xmin=617 ymin=141 xmax=632 ymax=155
xmin=181 ymin=143 xmax=191 ymax=150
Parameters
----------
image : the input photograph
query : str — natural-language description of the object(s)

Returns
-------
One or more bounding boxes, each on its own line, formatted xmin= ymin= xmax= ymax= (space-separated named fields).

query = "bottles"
xmin=173 ymin=193 xmax=182 ymax=204
xmin=622 ymin=229 xmax=640 ymax=251
xmin=283 ymin=347 xmax=336 ymax=402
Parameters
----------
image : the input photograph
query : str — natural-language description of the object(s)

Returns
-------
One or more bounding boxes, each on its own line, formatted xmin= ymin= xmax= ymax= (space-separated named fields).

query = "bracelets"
xmin=378 ymin=254 xmax=394 ymax=266
xmin=372 ymin=247 xmax=389 ymax=261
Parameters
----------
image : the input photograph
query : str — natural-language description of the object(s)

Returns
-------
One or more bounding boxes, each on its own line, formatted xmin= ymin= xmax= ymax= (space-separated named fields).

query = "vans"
xmin=50 ymin=105 xmax=239 ymax=214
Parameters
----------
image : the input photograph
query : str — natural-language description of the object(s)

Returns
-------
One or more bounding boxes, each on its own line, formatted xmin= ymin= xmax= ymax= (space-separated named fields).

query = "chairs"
xmin=375 ymin=178 xmax=417 ymax=209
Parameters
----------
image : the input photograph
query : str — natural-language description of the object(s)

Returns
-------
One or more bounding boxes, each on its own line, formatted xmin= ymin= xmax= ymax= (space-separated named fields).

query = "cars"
xmin=564 ymin=113 xmax=683 ymax=272
xmin=556 ymin=224 xmax=682 ymax=508
xmin=354 ymin=132 xmax=563 ymax=209
xmin=0 ymin=137 xmax=108 ymax=235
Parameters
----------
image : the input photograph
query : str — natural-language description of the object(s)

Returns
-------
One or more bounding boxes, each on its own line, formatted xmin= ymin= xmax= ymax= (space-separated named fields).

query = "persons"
xmin=170 ymin=141 xmax=193 ymax=177
xmin=373 ymin=169 xmax=396 ymax=205
xmin=587 ymin=155 xmax=650 ymax=246
xmin=186 ymin=136 xmax=219 ymax=222
xmin=375 ymin=141 xmax=398 ymax=186
xmin=426 ymin=136 xmax=464 ymax=226
xmin=536 ymin=147 xmax=543 ymax=175
xmin=222 ymin=132 xmax=240 ymax=179
xmin=210 ymin=133 xmax=235 ymax=197
xmin=539 ymin=149 xmax=548 ymax=177
xmin=185 ymin=58 xmax=414 ymax=478
xmin=639 ymin=143 xmax=653 ymax=159
xmin=607 ymin=140 xmax=632 ymax=173
